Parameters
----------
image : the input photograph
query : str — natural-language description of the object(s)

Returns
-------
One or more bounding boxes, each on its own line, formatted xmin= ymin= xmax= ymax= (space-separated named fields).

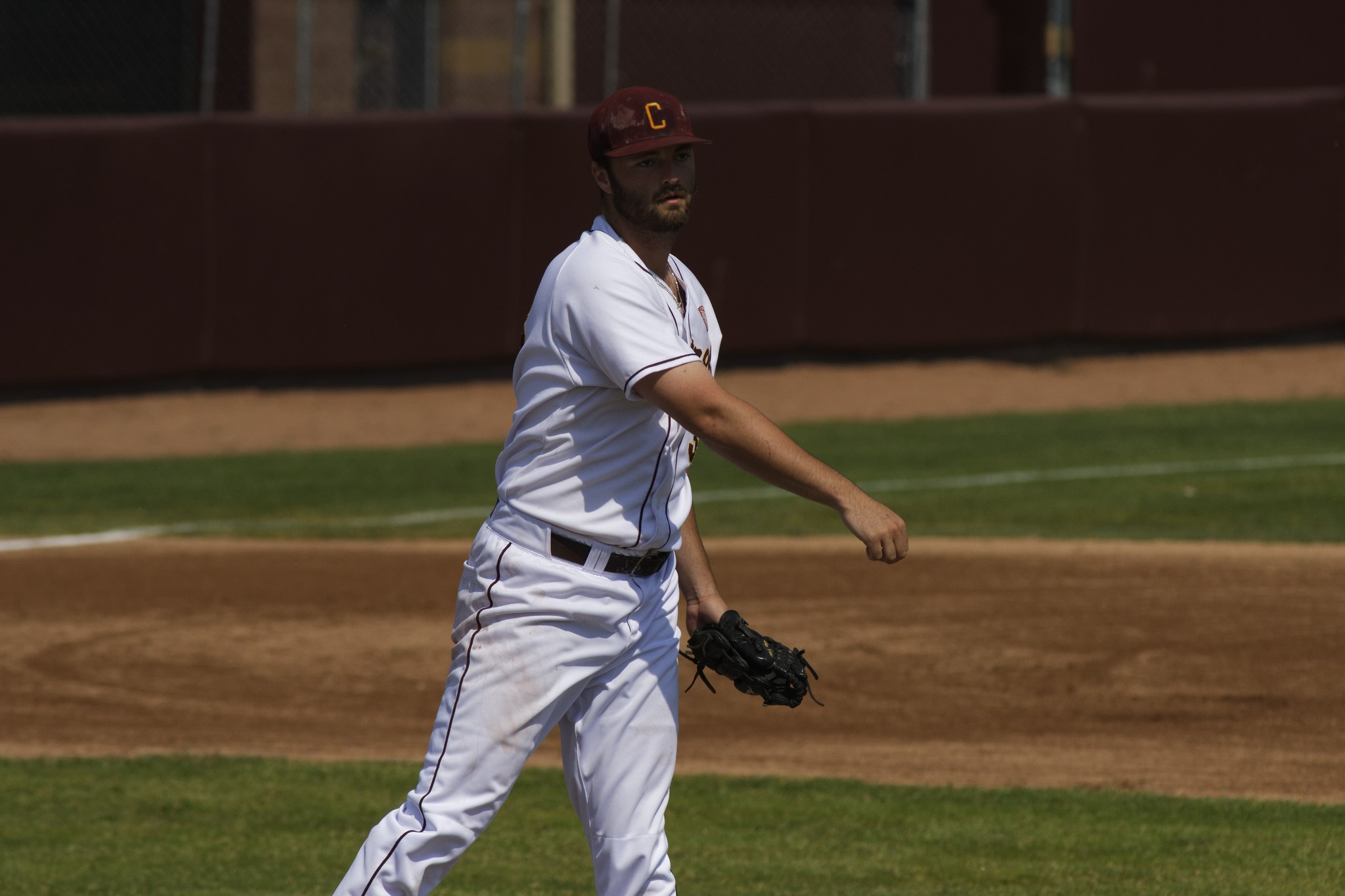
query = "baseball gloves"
xmin=685 ymin=608 xmax=810 ymax=709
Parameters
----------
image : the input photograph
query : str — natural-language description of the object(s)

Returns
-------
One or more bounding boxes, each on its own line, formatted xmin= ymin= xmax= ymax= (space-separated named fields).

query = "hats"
xmin=589 ymin=87 xmax=714 ymax=158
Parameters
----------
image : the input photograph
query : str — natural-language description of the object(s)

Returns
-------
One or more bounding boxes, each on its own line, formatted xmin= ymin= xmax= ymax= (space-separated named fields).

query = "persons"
xmin=332 ymin=86 xmax=907 ymax=896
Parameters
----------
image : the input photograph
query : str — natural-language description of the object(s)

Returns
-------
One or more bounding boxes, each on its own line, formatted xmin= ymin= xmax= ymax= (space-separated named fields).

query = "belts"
xmin=548 ymin=532 xmax=670 ymax=578
